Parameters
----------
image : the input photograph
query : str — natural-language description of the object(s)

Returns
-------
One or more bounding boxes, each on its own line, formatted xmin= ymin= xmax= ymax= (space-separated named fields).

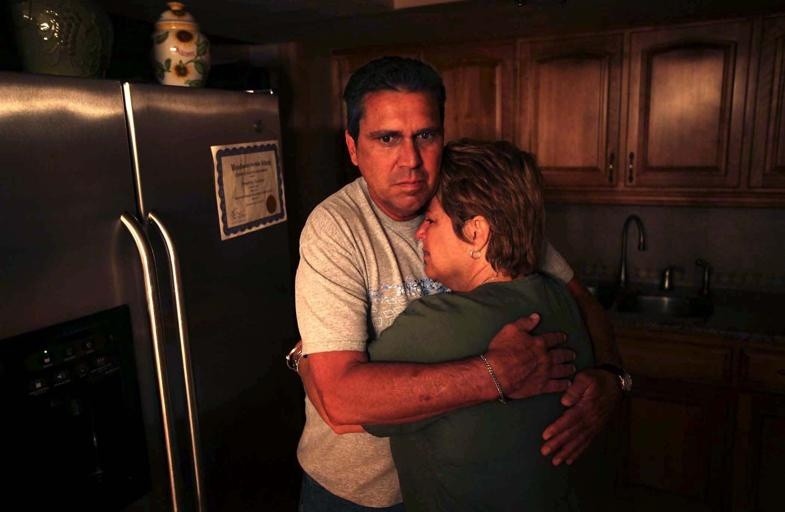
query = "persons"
xmin=294 ymin=55 xmax=637 ymax=510
xmin=280 ymin=135 xmax=617 ymax=510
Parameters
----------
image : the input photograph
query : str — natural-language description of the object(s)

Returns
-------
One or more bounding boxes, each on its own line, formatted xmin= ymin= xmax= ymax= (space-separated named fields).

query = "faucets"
xmin=696 ymin=257 xmax=714 ymax=297
xmin=619 ymin=214 xmax=647 ymax=291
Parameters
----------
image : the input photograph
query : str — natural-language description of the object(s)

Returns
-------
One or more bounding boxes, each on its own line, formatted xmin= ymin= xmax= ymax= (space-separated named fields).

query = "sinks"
xmin=607 ymin=287 xmax=722 ymax=327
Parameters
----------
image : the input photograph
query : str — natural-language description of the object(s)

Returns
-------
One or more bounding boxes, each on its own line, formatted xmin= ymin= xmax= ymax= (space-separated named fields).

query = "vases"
xmin=11 ymin=0 xmax=111 ymax=76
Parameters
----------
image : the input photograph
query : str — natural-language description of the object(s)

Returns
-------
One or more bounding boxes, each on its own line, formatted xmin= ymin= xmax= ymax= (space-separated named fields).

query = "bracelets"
xmin=285 ymin=347 xmax=304 ymax=374
xmin=478 ymin=354 xmax=508 ymax=405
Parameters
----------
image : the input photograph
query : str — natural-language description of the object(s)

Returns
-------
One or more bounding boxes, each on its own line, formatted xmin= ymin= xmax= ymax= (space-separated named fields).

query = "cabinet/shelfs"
xmin=583 ymin=320 xmax=785 ymax=512
xmin=291 ymin=0 xmax=785 ymax=211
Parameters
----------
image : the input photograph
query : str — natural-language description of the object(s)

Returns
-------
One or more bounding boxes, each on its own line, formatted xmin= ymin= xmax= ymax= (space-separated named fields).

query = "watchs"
xmin=598 ymin=362 xmax=633 ymax=401
